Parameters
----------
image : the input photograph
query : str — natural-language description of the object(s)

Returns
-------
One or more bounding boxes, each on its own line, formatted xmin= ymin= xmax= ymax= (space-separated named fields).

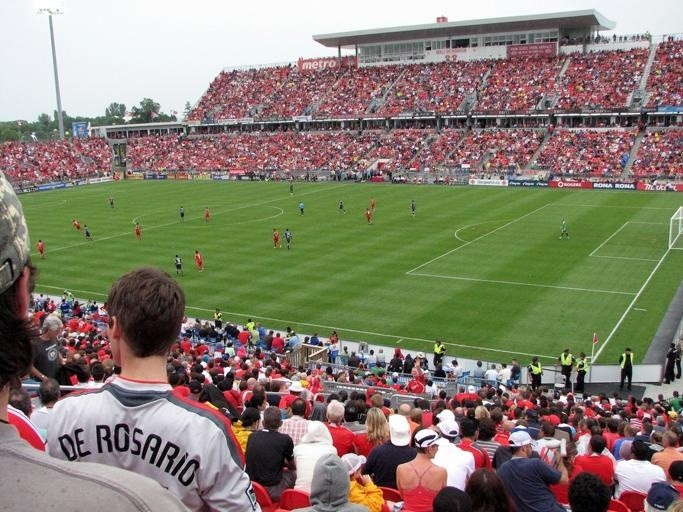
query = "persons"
xmin=125 ymin=29 xmax=683 ymax=180
xmin=1 ymin=135 xmax=113 ymax=190
xmin=2 ymin=172 xmax=682 ymax=511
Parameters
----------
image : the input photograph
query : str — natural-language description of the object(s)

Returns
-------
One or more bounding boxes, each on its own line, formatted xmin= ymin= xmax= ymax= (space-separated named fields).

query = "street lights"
xmin=35 ymin=5 xmax=65 ymax=139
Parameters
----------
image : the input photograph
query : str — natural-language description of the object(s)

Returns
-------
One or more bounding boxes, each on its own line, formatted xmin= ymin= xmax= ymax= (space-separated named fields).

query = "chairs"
xmin=1 ymin=129 xmax=196 ymax=178
xmin=195 ymin=46 xmax=682 ymax=178
xmin=0 ymin=303 xmax=683 ymax=512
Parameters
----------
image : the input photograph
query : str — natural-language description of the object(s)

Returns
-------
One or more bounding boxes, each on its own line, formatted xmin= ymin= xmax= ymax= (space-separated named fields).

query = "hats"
xmin=1 ymin=170 xmax=31 ymax=296
xmin=647 ymin=480 xmax=680 ymax=510
xmin=367 ymin=388 xmax=375 ymax=396
xmin=389 ymin=414 xmax=411 ymax=447
xmin=341 ymin=453 xmax=367 ymax=476
xmin=508 ymin=431 xmax=535 ymax=446
xmin=435 ymin=409 xmax=460 ymax=437
xmin=289 ymin=381 xmax=304 ymax=392
xmin=669 ymin=460 xmax=682 ymax=480
xmin=543 ymin=414 xmax=560 ymax=425
xmin=415 ymin=429 xmax=440 ymax=447
xmin=603 ymin=404 xmax=611 ymax=409
xmin=667 ymin=411 xmax=678 ymax=419
